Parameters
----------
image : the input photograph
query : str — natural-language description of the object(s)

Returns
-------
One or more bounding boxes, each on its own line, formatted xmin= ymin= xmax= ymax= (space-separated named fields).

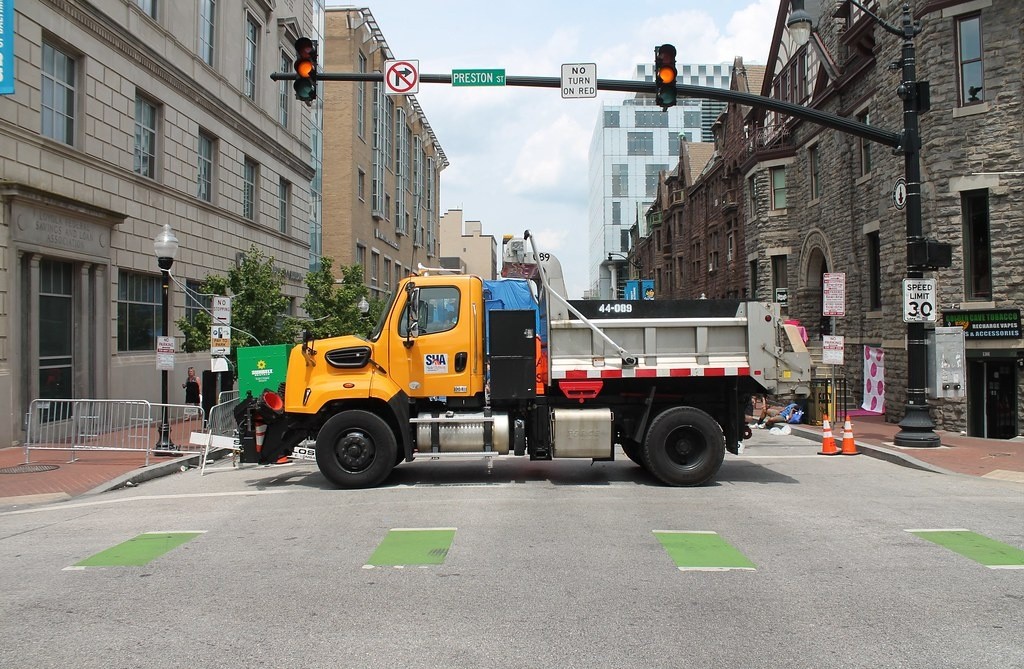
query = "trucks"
xmin=281 ymin=228 xmax=812 ymax=489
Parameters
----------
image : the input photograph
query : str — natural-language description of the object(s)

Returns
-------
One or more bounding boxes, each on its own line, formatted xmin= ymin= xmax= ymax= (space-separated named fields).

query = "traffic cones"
xmin=252 ymin=388 xmax=284 ymax=453
xmin=841 ymin=415 xmax=861 ymax=456
xmin=817 ymin=414 xmax=842 ymax=456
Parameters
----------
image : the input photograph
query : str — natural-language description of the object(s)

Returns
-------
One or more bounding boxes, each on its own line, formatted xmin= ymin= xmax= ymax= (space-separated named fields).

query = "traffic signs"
xmin=451 ymin=69 xmax=506 ymax=86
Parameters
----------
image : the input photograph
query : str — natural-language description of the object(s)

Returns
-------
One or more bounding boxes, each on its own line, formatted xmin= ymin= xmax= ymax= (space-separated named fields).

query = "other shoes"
xmin=750 ymin=423 xmax=759 ymax=429
xmin=198 ymin=415 xmax=202 ymax=420
xmin=758 ymin=423 xmax=766 ymax=429
xmin=184 ymin=417 xmax=189 ymax=420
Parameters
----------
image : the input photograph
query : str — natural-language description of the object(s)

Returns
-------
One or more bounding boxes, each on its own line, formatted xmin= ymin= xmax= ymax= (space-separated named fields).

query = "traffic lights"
xmin=295 ymin=37 xmax=317 ymax=101
xmin=911 ymin=241 xmax=952 ymax=267
xmin=655 ymin=44 xmax=678 ymax=107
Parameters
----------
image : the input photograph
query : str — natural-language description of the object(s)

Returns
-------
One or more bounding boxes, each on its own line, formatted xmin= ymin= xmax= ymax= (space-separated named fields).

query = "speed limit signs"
xmin=902 ymin=278 xmax=937 ymax=322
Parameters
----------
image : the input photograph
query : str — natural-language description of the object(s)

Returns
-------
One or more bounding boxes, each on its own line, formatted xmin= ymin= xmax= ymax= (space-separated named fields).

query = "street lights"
xmin=358 ymin=296 xmax=369 ymax=322
xmin=154 ymin=223 xmax=179 ymax=457
xmin=787 ymin=0 xmax=942 ymax=448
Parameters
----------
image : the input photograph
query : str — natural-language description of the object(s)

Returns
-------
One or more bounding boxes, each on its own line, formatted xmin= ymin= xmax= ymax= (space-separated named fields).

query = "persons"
xmin=421 ymin=300 xmax=458 ymax=332
xmin=182 ymin=367 xmax=203 ymax=421
xmin=747 ymin=393 xmax=800 ymax=429
